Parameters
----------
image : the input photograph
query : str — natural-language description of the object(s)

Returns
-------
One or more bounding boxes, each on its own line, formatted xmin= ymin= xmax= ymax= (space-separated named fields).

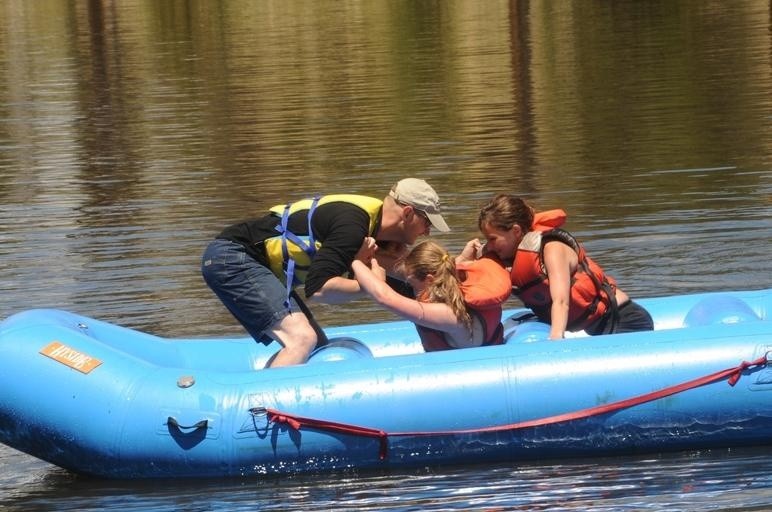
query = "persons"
xmin=474 ymin=193 xmax=653 ymax=341
xmin=202 ymin=177 xmax=479 ymax=367
xmin=350 ymin=236 xmax=504 ymax=352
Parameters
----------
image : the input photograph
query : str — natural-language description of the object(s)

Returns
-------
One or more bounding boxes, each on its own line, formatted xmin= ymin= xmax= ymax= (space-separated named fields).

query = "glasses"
xmin=395 ymin=199 xmax=432 ymax=228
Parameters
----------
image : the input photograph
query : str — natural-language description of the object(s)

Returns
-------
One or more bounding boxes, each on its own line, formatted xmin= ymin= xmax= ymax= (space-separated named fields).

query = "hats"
xmin=389 ymin=178 xmax=452 ymax=232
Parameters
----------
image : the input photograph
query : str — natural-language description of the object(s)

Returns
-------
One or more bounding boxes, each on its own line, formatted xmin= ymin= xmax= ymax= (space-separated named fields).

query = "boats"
xmin=0 ymin=287 xmax=771 ymax=481
